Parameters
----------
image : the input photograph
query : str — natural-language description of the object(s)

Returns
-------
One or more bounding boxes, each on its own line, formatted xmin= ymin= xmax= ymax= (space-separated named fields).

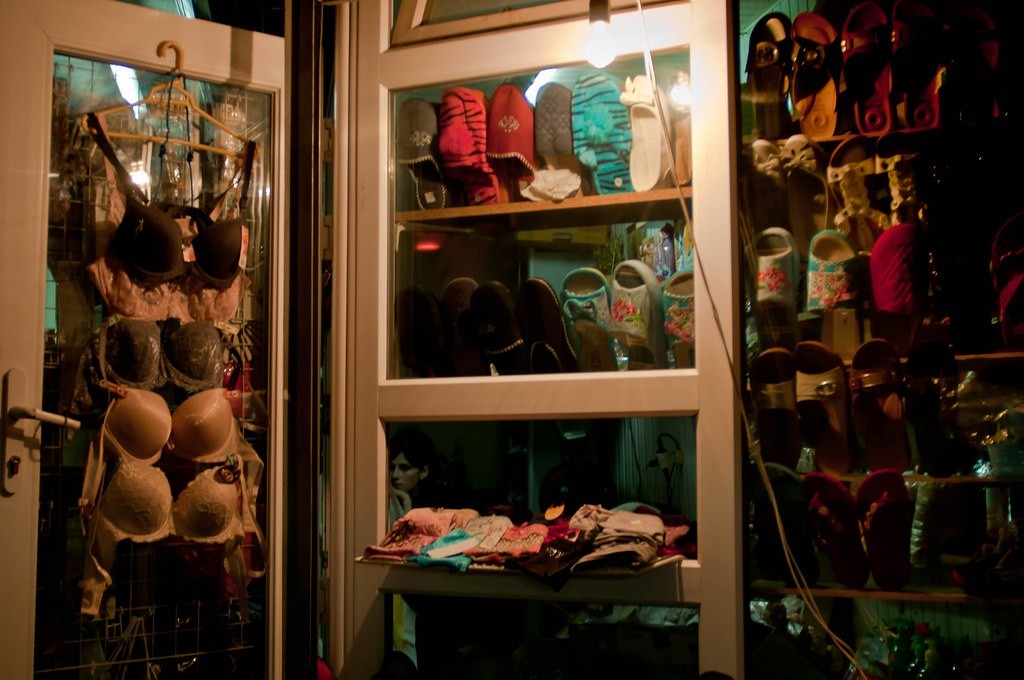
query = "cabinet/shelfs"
xmin=740 ymin=108 xmax=1023 ymax=606
xmin=375 ymin=186 xmax=701 ymax=605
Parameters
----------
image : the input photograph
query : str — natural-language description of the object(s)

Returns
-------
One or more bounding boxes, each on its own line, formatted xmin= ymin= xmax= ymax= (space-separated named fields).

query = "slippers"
xmin=396 ymin=69 xmax=692 ymax=212
xmin=742 ymin=0 xmax=1024 ymax=598
xmin=394 ymin=259 xmax=696 ymax=377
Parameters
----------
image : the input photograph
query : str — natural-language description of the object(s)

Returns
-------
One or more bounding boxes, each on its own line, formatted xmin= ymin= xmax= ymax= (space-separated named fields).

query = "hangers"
xmin=82 ymin=41 xmax=260 ymax=162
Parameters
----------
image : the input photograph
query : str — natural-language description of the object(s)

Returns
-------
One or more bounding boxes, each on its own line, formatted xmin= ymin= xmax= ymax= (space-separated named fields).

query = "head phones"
xmin=656 ymin=433 xmax=684 ymax=470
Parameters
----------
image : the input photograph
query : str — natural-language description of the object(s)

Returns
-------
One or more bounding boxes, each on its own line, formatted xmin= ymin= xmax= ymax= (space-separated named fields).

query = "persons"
xmin=386 ymin=425 xmax=449 ymax=680
